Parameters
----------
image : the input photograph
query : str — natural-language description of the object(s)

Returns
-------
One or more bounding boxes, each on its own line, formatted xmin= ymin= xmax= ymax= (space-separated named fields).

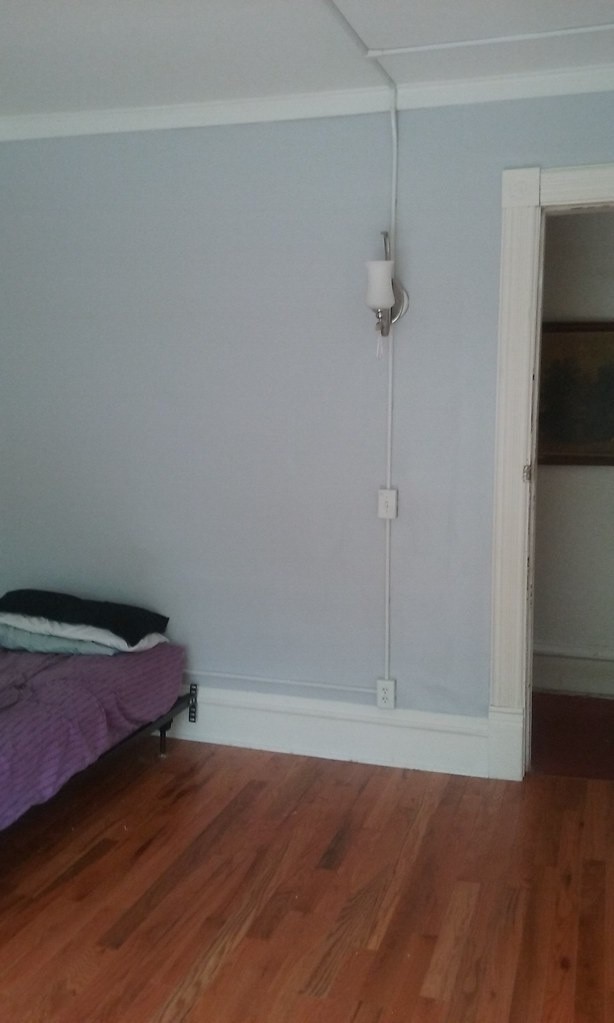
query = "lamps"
xmin=365 ymin=259 xmax=401 ymax=339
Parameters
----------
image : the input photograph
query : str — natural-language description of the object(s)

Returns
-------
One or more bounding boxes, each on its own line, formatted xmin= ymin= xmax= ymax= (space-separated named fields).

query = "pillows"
xmin=0 ymin=613 xmax=170 ymax=652
xmin=0 ymin=589 xmax=167 ymax=648
xmin=0 ymin=625 xmax=119 ymax=656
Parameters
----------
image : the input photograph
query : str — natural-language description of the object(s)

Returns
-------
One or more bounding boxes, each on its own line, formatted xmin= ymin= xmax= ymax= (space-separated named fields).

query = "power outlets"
xmin=376 ymin=679 xmax=397 ymax=710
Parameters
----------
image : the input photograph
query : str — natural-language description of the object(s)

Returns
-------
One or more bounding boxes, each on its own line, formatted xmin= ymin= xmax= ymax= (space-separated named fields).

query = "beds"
xmin=0 ymin=586 xmax=197 ymax=835
